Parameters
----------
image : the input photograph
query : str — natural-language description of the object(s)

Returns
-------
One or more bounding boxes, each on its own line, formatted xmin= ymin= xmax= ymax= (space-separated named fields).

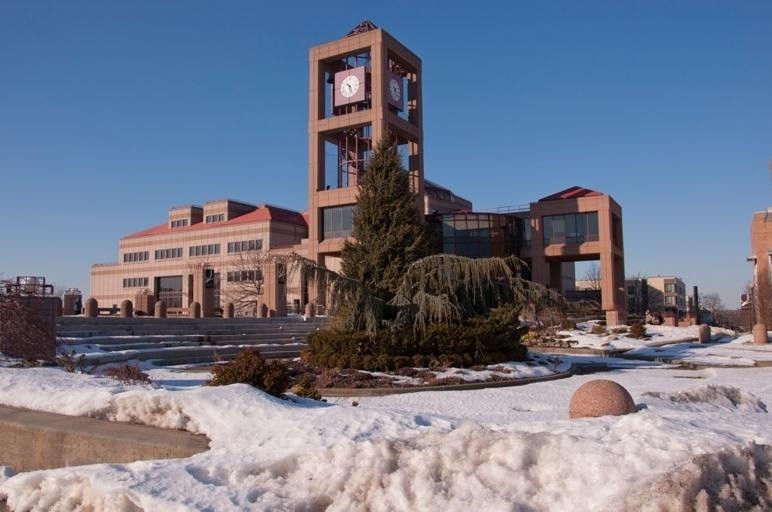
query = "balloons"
xmin=389 ymin=79 xmax=400 ymax=102
xmin=340 ymin=75 xmax=359 ymax=97
